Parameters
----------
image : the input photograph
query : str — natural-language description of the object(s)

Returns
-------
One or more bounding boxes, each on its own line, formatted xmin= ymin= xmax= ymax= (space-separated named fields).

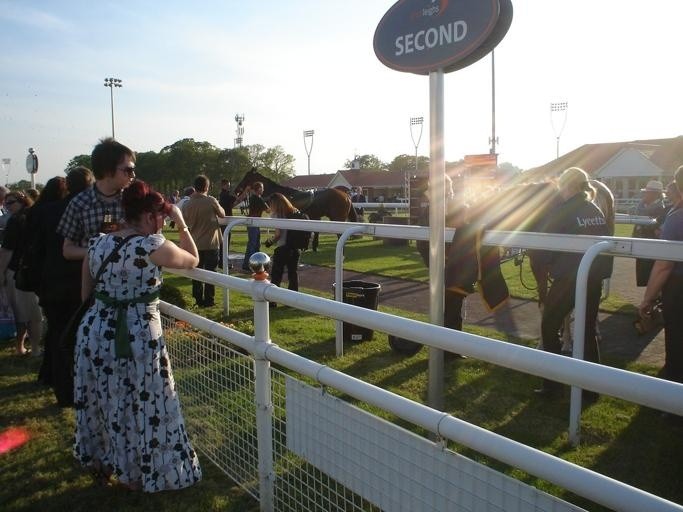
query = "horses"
xmin=464 ymin=178 xmax=619 ymax=353
xmin=233 ymin=165 xmax=358 ymax=256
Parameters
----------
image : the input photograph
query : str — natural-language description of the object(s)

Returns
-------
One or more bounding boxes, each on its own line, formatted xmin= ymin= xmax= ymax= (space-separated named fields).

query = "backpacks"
xmin=15 ymin=232 xmax=47 ymax=294
xmin=284 ymin=209 xmax=311 ymax=250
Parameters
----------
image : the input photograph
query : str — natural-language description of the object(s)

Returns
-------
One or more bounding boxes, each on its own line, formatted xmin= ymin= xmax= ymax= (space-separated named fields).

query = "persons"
xmin=416 ymin=166 xmax=682 ymax=422
xmin=351 ymin=186 xmax=409 ymax=223
xmin=163 ymin=174 xmax=300 ymax=309
xmin=0 ymin=167 xmax=96 ymax=407
xmin=55 ymin=137 xmax=136 ymax=260
xmin=73 ymin=177 xmax=202 ymax=493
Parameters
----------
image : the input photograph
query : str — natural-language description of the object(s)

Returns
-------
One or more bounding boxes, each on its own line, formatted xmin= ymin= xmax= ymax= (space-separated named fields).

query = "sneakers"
xmin=194 ymin=298 xmax=214 ymax=307
xmin=217 ymin=259 xmax=269 ymax=272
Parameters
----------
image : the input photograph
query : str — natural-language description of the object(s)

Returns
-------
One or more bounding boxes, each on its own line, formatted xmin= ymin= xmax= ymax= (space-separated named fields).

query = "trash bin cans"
xmin=332 ymin=280 xmax=381 ymax=343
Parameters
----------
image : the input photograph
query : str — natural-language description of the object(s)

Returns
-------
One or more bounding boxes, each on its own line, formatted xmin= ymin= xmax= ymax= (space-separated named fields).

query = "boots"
xmin=577 ymin=333 xmax=605 ymax=400
xmin=533 ymin=328 xmax=563 ymax=399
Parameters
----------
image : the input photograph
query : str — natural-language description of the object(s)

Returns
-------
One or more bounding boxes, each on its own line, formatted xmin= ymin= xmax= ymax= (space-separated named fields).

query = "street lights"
xmin=302 ymin=130 xmax=314 ymax=175
xmin=103 ymin=76 xmax=124 ymax=141
xmin=408 ymin=117 xmax=424 ymax=169
xmin=0 ymin=158 xmax=12 ymax=189
xmin=545 ymin=101 xmax=569 ymax=160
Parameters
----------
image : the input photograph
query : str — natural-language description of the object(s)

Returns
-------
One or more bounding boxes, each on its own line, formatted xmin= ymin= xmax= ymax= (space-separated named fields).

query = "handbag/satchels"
xmin=59 ymin=294 xmax=93 ymax=350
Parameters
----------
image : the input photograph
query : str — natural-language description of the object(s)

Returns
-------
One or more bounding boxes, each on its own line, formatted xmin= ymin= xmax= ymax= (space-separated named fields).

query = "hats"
xmin=555 ymin=166 xmax=589 ymax=190
xmin=640 ymin=180 xmax=668 ymax=195
xmin=423 ymin=171 xmax=454 ymax=201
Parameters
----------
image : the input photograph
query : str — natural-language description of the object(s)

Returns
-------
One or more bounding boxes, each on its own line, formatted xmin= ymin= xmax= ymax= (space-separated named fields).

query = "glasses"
xmin=3 ymin=199 xmax=20 ymax=207
xmin=115 ymin=165 xmax=138 ymax=176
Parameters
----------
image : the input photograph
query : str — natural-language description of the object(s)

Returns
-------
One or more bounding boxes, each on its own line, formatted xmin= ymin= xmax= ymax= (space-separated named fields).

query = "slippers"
xmin=15 ymin=348 xmax=45 ymax=357
